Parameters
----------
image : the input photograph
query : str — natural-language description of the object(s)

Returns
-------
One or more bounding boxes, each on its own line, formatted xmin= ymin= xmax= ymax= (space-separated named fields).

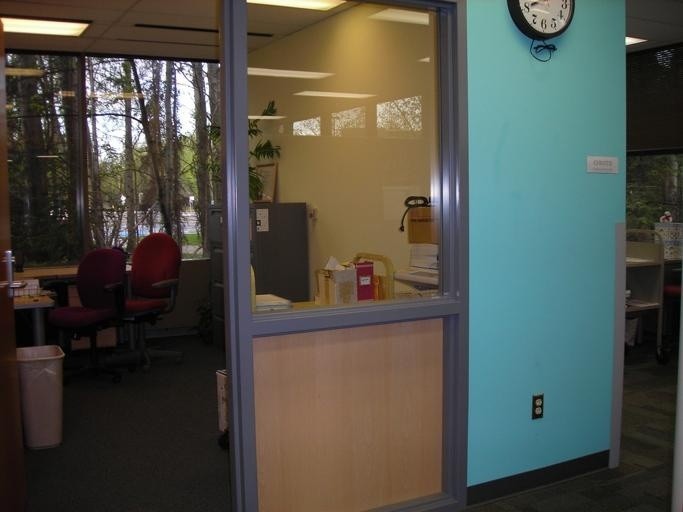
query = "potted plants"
xmin=192 ymin=295 xmax=217 ymax=343
xmin=204 ymin=101 xmax=280 ymax=209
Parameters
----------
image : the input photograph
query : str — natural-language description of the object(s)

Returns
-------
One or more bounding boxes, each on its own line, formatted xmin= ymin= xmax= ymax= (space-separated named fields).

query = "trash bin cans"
xmin=16 ymin=345 xmax=65 ymax=447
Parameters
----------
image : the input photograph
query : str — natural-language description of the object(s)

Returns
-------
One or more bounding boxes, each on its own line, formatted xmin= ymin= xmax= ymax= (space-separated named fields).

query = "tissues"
xmin=315 ymin=255 xmax=358 ymax=307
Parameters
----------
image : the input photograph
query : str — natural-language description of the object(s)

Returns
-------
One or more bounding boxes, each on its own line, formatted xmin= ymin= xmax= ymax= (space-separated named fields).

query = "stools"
xmin=663 ymin=284 xmax=681 ymax=356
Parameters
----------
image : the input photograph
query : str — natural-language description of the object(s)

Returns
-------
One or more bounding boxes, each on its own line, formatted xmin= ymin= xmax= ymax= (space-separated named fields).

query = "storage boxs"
xmin=216 ymin=369 xmax=229 ymax=432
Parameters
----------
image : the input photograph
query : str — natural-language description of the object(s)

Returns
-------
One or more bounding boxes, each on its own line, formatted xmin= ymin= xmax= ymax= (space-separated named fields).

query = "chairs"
xmin=48 ymin=248 xmax=126 ymax=386
xmin=124 ymin=233 xmax=181 ymax=373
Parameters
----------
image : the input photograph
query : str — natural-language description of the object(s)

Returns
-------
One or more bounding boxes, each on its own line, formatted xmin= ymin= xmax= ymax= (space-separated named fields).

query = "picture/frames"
xmin=254 ymin=163 xmax=278 ymax=203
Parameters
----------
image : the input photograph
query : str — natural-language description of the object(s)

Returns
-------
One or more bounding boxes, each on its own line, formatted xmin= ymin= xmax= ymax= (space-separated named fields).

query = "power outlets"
xmin=532 ymin=394 xmax=544 ymax=420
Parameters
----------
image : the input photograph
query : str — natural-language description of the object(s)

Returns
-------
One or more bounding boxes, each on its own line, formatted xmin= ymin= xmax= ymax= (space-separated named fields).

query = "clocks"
xmin=507 ymin=0 xmax=575 ymax=41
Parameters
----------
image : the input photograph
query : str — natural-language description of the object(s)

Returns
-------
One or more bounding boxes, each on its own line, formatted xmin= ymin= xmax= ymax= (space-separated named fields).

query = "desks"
xmin=13 ymin=265 xmax=137 ymax=352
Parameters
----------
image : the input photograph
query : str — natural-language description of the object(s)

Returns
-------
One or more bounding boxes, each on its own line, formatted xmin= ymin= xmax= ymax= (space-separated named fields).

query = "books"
xmin=254 ymin=295 xmax=293 ymax=313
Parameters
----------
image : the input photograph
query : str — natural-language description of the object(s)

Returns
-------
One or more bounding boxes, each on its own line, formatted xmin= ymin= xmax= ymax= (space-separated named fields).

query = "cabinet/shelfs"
xmin=207 ymin=202 xmax=309 ymax=362
xmin=624 ymin=229 xmax=665 ymax=355
